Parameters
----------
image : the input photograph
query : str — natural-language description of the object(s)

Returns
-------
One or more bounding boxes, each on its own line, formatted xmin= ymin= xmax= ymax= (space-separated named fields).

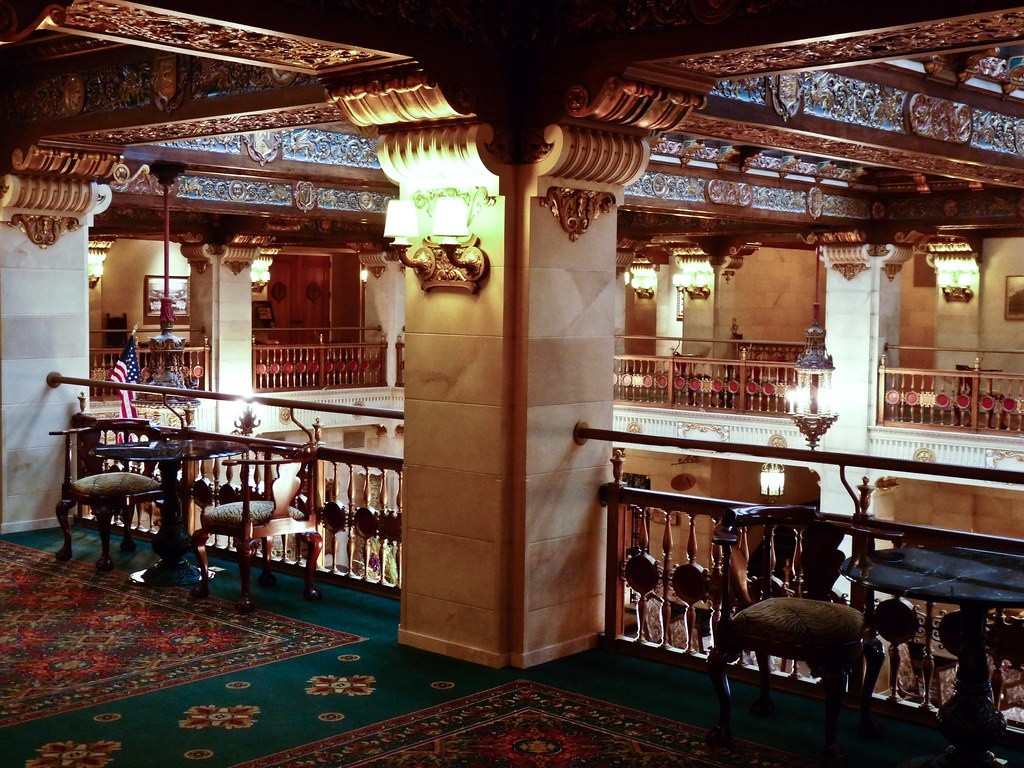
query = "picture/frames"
xmin=145 ymin=275 xmax=190 ymax=317
xmin=1004 ymin=275 xmax=1024 ymax=321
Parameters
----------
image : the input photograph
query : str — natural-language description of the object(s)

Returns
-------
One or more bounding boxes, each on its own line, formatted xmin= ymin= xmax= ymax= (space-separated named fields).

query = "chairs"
xmin=49 ymin=418 xmax=162 ymax=573
xmin=707 ymin=503 xmax=904 ymax=768
xmin=190 ymin=452 xmax=323 ymax=614
xmin=954 ymin=364 xmax=1008 ymax=429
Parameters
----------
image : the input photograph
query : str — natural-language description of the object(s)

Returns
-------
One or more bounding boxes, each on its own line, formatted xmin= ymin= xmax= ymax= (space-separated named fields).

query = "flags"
xmin=106 ymin=334 xmax=142 ymax=444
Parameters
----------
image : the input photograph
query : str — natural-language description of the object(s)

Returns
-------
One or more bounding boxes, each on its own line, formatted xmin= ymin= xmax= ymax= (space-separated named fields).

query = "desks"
xmin=838 ymin=547 xmax=1024 ymax=768
xmin=88 ymin=441 xmax=249 ymax=587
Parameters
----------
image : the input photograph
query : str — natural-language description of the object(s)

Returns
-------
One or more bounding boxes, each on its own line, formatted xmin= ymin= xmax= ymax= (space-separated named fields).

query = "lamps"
xmin=250 ymin=263 xmax=271 ymax=292
xmin=384 ymin=196 xmax=490 ymax=295
xmin=681 ymin=274 xmax=711 ymax=301
xmin=88 ymin=265 xmax=104 ymax=288
xmin=760 ymin=463 xmax=786 ymax=504
xmin=790 ymin=236 xmax=839 ymax=450
xmin=631 ymin=278 xmax=654 ymax=300
xmin=131 ymin=161 xmax=201 ymax=428
xmin=938 ymin=273 xmax=974 ymax=304
xmin=673 ymin=273 xmax=684 ymax=294
